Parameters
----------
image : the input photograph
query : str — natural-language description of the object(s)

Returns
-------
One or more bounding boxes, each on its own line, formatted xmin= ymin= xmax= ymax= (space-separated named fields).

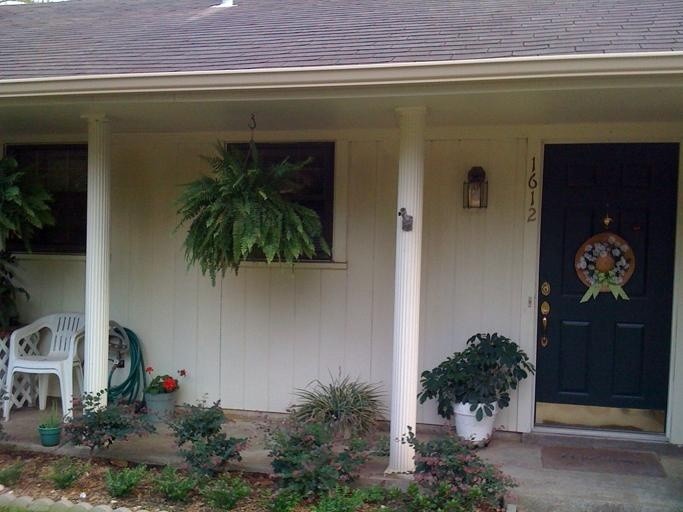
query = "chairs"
xmin=2 ymin=311 xmax=85 ymax=424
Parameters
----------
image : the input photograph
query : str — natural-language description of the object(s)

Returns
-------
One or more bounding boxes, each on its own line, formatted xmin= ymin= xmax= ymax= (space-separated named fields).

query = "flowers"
xmin=141 ymin=367 xmax=186 ymax=394
xmin=576 ymin=241 xmax=631 ymax=304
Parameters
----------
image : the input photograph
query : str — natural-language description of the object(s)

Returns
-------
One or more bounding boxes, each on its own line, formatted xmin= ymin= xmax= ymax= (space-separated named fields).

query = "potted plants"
xmin=417 ymin=332 xmax=536 ymax=449
xmin=541 ymin=446 xmax=668 ymax=479
xmin=290 ymin=379 xmax=388 ymax=442
xmin=169 ymin=135 xmax=331 ymax=287
xmin=37 ymin=400 xmax=64 ymax=447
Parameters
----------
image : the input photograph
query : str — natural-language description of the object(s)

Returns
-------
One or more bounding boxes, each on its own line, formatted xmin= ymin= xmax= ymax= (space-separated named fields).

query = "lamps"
xmin=462 ymin=166 xmax=488 ymax=209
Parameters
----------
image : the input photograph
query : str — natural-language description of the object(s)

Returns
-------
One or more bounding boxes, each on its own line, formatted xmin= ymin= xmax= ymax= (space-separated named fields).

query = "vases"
xmin=145 ymin=390 xmax=177 ymax=423
